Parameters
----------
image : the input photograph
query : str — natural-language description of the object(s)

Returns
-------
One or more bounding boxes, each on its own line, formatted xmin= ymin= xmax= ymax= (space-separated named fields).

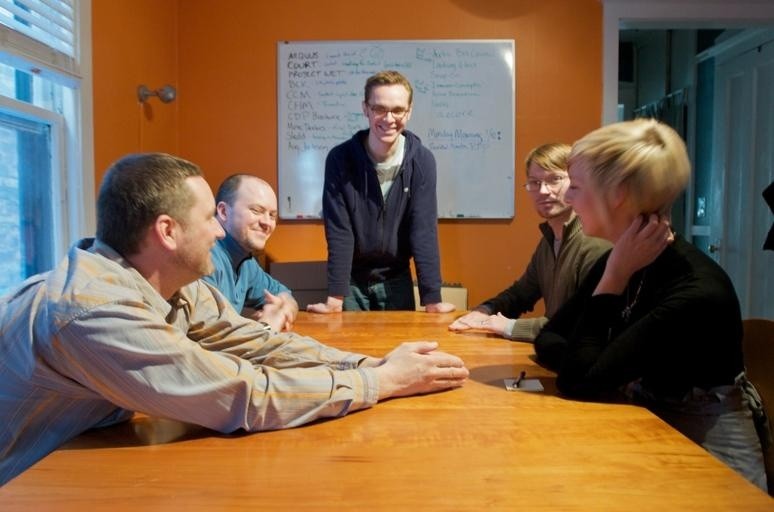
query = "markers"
xmin=296 ymin=214 xmax=319 ymax=219
xmin=450 ymin=213 xmax=480 ymax=218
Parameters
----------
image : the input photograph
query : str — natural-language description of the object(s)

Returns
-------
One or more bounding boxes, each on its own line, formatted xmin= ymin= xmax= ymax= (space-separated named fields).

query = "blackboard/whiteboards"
xmin=277 ymin=39 xmax=516 ymax=221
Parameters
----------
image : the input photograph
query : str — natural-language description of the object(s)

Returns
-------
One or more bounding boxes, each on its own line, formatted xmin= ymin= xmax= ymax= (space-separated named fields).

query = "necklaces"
xmin=621 ymin=269 xmax=647 ymax=322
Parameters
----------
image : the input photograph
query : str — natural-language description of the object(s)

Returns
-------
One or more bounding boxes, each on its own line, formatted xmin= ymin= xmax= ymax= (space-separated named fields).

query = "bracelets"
xmin=259 ymin=322 xmax=270 ymax=330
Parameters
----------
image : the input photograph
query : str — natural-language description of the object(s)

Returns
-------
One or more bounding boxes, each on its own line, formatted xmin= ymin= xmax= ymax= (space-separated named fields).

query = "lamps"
xmin=137 ymin=84 xmax=175 ymax=105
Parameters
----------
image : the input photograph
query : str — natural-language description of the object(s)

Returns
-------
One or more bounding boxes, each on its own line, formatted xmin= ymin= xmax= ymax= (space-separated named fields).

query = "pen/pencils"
xmin=513 ymin=370 xmax=526 ymax=388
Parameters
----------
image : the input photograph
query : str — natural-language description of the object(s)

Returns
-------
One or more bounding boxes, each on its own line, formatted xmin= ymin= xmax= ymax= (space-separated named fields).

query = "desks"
xmin=0 ymin=310 xmax=773 ymax=511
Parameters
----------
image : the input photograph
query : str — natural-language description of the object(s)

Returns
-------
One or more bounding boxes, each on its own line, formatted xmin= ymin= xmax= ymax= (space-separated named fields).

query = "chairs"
xmin=269 ymin=260 xmax=340 ymax=310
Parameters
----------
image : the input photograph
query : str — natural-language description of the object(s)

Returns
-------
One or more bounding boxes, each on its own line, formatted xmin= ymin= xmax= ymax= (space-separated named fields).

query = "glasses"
xmin=523 ymin=175 xmax=565 ymax=192
xmin=365 ymin=102 xmax=409 ymax=120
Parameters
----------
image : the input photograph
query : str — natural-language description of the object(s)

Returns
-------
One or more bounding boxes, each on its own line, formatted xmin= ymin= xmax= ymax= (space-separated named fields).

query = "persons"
xmin=200 ymin=172 xmax=299 ymax=334
xmin=0 ymin=152 xmax=470 ymax=488
xmin=533 ymin=118 xmax=768 ymax=494
xmin=306 ymin=70 xmax=457 ymax=315
xmin=448 ymin=141 xmax=613 ymax=343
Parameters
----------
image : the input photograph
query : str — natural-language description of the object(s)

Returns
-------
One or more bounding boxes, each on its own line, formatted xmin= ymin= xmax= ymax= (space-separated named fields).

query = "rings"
xmin=481 ymin=321 xmax=484 ymax=325
